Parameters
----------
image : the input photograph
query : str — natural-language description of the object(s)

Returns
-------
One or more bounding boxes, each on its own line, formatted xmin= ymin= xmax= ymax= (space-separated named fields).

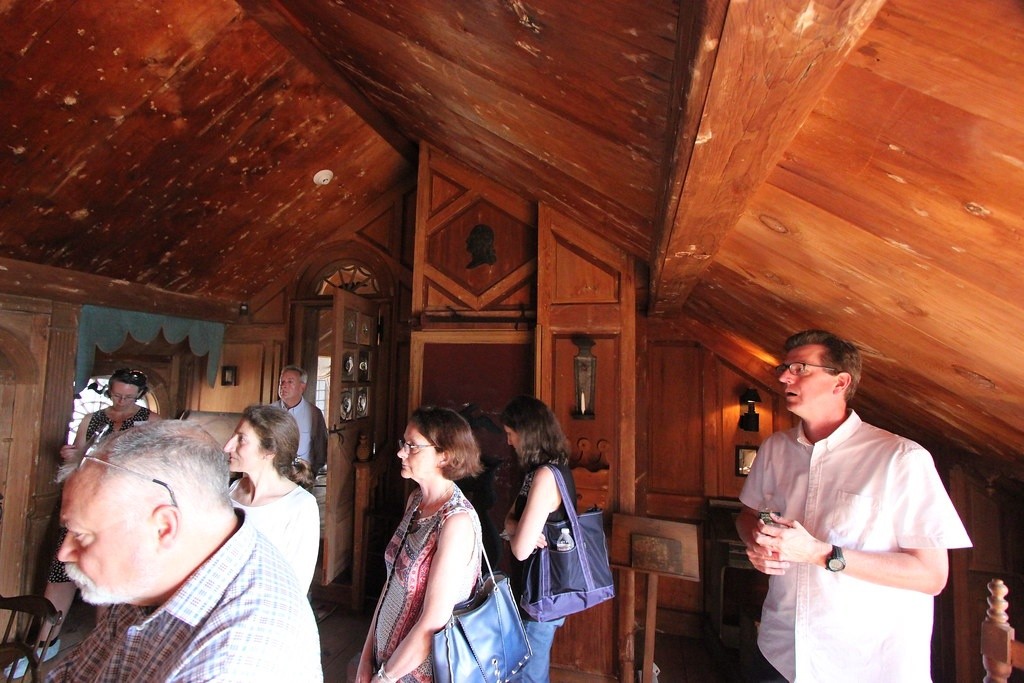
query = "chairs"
xmin=0 ymin=594 xmax=62 ymax=683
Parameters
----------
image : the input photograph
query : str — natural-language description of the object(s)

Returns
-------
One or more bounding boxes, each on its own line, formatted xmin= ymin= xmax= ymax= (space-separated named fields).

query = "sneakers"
xmin=4 ymin=639 xmax=61 ymax=679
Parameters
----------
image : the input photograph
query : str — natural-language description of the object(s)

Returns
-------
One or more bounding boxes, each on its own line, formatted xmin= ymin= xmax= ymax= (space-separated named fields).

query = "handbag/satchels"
xmin=431 ymin=508 xmax=532 ymax=683
xmin=519 ymin=463 xmax=615 ymax=622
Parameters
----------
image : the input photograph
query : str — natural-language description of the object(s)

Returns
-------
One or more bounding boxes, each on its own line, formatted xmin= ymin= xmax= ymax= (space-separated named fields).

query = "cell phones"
xmin=767 ymin=522 xmax=789 ymax=530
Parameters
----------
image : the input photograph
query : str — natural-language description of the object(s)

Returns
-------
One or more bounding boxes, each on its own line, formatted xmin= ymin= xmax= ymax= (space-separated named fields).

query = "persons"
xmin=39 ymin=419 xmax=324 ymax=683
xmin=265 ymin=365 xmax=330 ymax=498
xmin=3 ymin=367 xmax=161 ymax=678
xmin=734 ymin=328 xmax=973 ymax=683
xmin=223 ymin=404 xmax=321 ymax=598
xmin=500 ymin=394 xmax=579 ymax=682
xmin=354 ymin=406 xmax=487 ymax=683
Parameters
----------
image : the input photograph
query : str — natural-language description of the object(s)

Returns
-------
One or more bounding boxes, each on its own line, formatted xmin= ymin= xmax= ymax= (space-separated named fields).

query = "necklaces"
xmin=416 ymin=480 xmax=454 ymax=514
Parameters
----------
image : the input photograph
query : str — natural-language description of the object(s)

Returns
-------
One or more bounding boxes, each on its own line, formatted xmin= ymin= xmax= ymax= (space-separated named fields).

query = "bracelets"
xmin=376 ymin=661 xmax=399 ymax=683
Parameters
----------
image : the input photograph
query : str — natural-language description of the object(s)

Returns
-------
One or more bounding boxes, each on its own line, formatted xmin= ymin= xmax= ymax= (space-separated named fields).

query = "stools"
xmin=736 ymin=601 xmax=766 ymax=683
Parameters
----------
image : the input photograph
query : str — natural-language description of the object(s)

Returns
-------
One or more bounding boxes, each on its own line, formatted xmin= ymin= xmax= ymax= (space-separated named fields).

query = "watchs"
xmin=824 ymin=544 xmax=846 ymax=572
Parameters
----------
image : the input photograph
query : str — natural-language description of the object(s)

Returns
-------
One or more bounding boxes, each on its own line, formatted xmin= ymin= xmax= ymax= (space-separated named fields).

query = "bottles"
xmin=758 ymin=492 xmax=782 ymax=536
xmin=556 ymin=527 xmax=574 ymax=552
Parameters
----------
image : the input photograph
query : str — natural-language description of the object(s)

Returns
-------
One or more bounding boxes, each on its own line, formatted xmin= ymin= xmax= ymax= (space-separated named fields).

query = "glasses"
xmin=775 ymin=362 xmax=839 ymax=375
xmin=123 ymin=367 xmax=143 ymax=379
xmin=398 ymin=439 xmax=436 ymax=455
xmin=75 ymin=423 xmax=178 ymax=508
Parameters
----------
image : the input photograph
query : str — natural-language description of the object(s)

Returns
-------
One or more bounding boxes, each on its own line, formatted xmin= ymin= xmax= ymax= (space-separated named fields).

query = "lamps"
xmin=739 ymin=388 xmax=761 ymax=432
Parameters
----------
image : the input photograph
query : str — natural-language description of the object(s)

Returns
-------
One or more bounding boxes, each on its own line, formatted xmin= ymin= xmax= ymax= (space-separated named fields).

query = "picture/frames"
xmin=220 ymin=364 xmax=237 ymax=386
xmin=735 ymin=445 xmax=760 ymax=477
